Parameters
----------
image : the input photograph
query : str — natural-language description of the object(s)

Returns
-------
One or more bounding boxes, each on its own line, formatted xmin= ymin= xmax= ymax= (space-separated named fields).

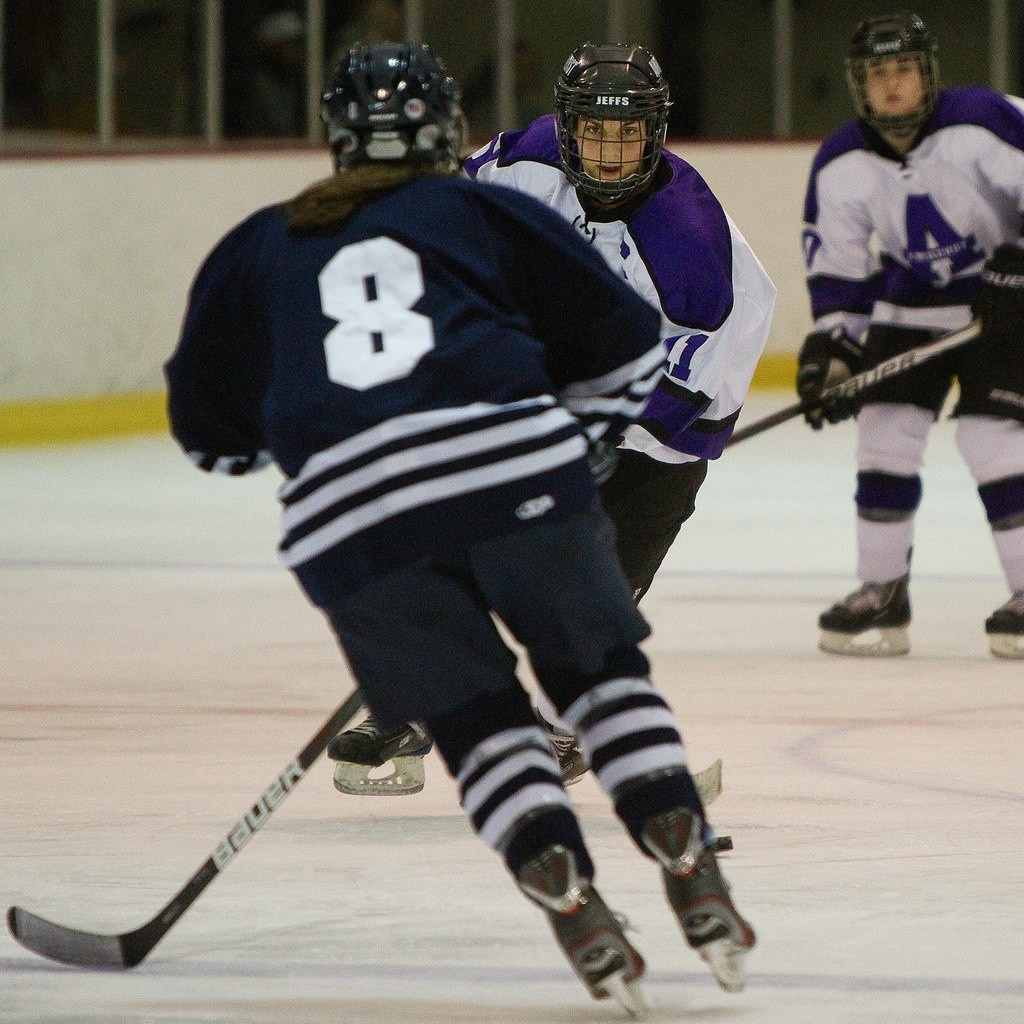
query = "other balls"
xmin=708 ymin=833 xmax=734 ymax=855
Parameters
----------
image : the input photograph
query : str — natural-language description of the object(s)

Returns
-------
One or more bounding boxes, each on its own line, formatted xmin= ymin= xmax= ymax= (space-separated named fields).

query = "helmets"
xmin=318 ymin=31 xmax=463 ymax=172
xmin=551 ymin=40 xmax=671 ymax=197
xmin=842 ymin=10 xmax=940 ymax=126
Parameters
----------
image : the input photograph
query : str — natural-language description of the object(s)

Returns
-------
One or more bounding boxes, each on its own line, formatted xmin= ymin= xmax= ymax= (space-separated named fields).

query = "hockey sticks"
xmin=4 ymin=683 xmax=368 ymax=977
xmin=688 ymin=757 xmax=728 ymax=810
xmin=719 ymin=315 xmax=987 ymax=455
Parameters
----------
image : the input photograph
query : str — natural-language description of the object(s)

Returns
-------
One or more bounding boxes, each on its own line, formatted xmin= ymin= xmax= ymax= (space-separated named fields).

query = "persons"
xmin=796 ymin=14 xmax=1023 ymax=635
xmin=165 ymin=34 xmax=775 ymax=999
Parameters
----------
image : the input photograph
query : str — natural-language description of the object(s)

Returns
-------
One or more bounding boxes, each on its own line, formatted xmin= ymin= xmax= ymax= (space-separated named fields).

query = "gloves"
xmin=796 ymin=329 xmax=868 ymax=432
xmin=971 ymin=241 xmax=1024 ymax=321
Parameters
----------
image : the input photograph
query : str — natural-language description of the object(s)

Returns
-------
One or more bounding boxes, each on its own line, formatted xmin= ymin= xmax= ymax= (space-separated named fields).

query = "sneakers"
xmin=816 ymin=583 xmax=915 ymax=662
xmin=981 ymin=590 xmax=1024 ymax=663
xmin=537 ymin=886 xmax=653 ymax=1018
xmin=660 ymin=858 xmax=759 ymax=993
xmin=539 ymin=708 xmax=590 ymax=788
xmin=327 ymin=713 xmax=432 ymax=798
